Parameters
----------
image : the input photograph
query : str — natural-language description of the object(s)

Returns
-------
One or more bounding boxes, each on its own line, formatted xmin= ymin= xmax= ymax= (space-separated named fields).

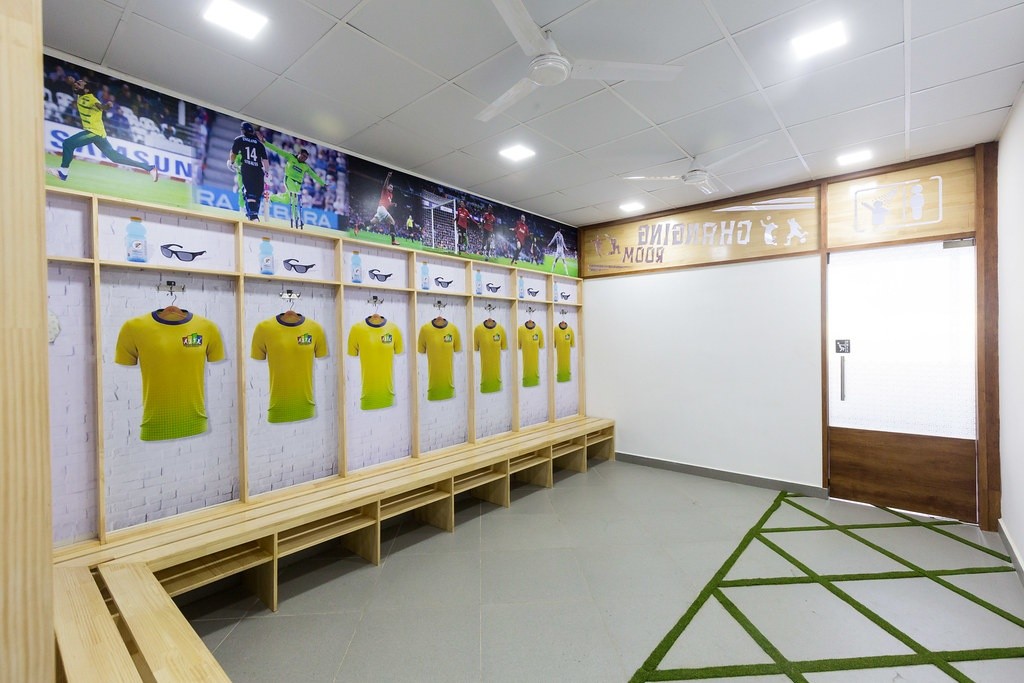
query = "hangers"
xmin=279 ymin=299 xmax=302 ymax=322
xmin=485 ymin=307 xmax=495 ymax=328
xmin=525 ymin=310 xmax=535 ymax=328
xmin=367 ymin=305 xmax=384 ymax=323
xmin=559 ymin=312 xmax=568 ymax=330
xmin=433 ymin=308 xmax=446 ymax=325
xmin=156 ymin=292 xmax=188 ymax=320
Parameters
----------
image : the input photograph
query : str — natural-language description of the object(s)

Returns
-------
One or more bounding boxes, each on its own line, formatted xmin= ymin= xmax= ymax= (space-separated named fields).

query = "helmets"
xmin=240 ymin=122 xmax=254 ymax=132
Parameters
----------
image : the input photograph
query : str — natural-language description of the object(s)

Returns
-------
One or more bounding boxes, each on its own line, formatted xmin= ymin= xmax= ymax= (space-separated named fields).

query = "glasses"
xmin=369 ymin=269 xmax=393 ymax=282
xmin=159 ymin=243 xmax=206 ymax=262
xmin=434 ymin=277 xmax=453 ymax=288
xmin=527 ymin=288 xmax=539 ymax=297
xmin=561 ymin=292 xmax=571 ymax=300
xmin=486 ymin=283 xmax=501 ymax=293
xmin=283 ymin=259 xmax=316 ymax=274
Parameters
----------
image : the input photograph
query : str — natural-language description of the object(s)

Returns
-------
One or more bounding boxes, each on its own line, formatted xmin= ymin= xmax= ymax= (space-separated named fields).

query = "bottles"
xmin=260 ymin=237 xmax=274 ymax=274
xmin=554 ymin=281 xmax=558 ymax=300
xmin=422 ymin=262 xmax=429 ymax=289
xmin=352 ymin=251 xmax=362 ymax=283
xmin=519 ymin=276 xmax=524 ymax=298
xmin=476 ymin=270 xmax=482 ymax=294
xmin=127 ymin=217 xmax=146 ymax=263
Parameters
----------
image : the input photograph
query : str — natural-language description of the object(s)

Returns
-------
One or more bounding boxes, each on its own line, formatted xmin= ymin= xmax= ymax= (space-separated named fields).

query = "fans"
xmin=623 ymin=142 xmax=763 ymax=195
xmin=475 ymin=0 xmax=682 ymax=124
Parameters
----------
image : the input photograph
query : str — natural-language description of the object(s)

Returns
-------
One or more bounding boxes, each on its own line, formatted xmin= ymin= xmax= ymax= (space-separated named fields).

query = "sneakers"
xmin=392 ymin=240 xmax=400 ymax=245
xmin=354 ymin=224 xmax=359 ymax=236
xmin=150 ymin=165 xmax=158 ymax=183
xmin=46 ymin=167 xmax=68 ymax=181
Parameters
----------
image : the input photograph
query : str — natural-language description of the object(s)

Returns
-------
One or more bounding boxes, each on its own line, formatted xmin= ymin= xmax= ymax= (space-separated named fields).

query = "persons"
xmin=228 ymin=122 xmax=271 ymax=221
xmin=511 ymin=214 xmax=529 ymax=266
xmin=453 ymin=200 xmax=480 ymax=251
xmin=407 ymin=215 xmax=415 ymax=242
xmin=193 ymin=106 xmax=208 ymax=151
xmin=44 ymin=65 xmax=173 ymax=150
xmin=530 ymin=238 xmax=541 ymax=265
xmin=46 ymin=77 xmax=159 ymax=182
xmin=253 ymin=124 xmax=345 ymax=215
xmin=548 ymin=229 xmax=569 ymax=276
xmin=482 ymin=204 xmax=496 ymax=261
xmin=350 ymin=176 xmax=577 ymax=262
xmin=259 ymin=136 xmax=328 ymax=229
xmin=354 ymin=171 xmax=400 ymax=245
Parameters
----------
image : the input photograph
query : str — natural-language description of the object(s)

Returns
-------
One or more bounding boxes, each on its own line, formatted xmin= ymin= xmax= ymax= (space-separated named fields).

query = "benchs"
xmin=52 ymin=416 xmax=617 ymax=683
